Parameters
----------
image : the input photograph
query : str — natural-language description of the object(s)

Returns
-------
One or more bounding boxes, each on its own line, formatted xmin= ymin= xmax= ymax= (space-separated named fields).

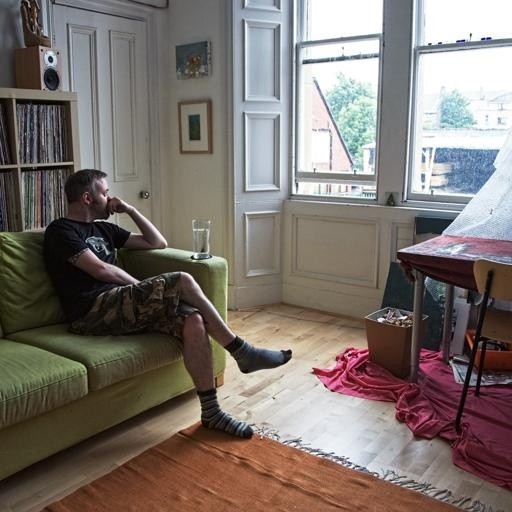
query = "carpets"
xmin=41 ymin=417 xmax=493 ymax=512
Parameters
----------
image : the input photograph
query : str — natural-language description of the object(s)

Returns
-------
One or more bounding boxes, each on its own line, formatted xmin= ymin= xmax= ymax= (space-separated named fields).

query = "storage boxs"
xmin=467 ymin=325 xmax=512 ymax=373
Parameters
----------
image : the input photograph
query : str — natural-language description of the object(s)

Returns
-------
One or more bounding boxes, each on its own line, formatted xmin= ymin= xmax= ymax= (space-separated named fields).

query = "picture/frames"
xmin=177 ymin=97 xmax=217 ymax=154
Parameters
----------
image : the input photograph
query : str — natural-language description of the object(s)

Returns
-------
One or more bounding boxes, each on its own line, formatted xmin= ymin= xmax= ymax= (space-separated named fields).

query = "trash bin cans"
xmin=364 ymin=307 xmax=431 ymax=379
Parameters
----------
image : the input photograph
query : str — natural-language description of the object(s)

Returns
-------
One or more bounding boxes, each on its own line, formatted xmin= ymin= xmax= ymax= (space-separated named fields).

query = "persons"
xmin=0 ymin=104 xmax=69 ymax=231
xmin=46 ymin=169 xmax=292 ymax=439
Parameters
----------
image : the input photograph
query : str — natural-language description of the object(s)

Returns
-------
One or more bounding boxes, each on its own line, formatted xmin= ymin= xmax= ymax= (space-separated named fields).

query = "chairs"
xmin=453 ymin=252 xmax=512 ymax=432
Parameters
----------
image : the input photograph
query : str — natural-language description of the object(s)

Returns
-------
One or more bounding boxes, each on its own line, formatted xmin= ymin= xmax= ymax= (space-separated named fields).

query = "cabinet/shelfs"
xmin=0 ymin=87 xmax=88 ymax=234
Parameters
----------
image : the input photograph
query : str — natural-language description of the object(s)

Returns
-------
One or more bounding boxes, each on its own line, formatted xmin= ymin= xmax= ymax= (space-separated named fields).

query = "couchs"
xmin=1 ymin=225 xmax=227 ymax=484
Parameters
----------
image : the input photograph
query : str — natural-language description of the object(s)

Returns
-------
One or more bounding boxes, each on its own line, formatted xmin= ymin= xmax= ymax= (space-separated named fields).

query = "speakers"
xmin=15 ymin=45 xmax=64 ymax=92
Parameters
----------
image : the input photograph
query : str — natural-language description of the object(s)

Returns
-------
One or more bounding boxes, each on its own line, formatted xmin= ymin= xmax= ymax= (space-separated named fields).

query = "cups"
xmin=191 ymin=218 xmax=212 ymax=260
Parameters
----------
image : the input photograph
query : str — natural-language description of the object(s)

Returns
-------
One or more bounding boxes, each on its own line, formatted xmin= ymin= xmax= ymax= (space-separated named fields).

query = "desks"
xmin=396 ymin=233 xmax=512 ymax=383
